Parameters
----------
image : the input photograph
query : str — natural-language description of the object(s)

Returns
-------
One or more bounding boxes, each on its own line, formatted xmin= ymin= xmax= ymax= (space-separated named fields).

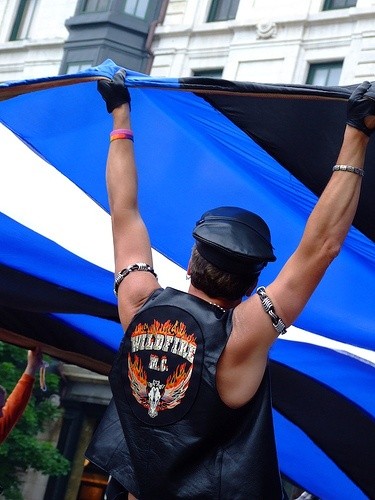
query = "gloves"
xmin=344 ymin=81 xmax=375 ymax=137
xmin=97 ymin=68 xmax=132 ymax=114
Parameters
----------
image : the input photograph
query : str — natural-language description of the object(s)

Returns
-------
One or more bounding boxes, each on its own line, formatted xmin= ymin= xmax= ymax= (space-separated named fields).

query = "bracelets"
xmin=109 ymin=129 xmax=134 ymax=141
xmin=332 ymin=165 xmax=364 ymax=177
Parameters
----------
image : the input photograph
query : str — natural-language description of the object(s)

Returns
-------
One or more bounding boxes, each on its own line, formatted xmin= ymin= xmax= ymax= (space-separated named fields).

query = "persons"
xmin=98 ymin=61 xmax=375 ymax=500
xmin=0 ymin=344 xmax=43 ymax=444
xmin=104 ymin=474 xmax=139 ymax=500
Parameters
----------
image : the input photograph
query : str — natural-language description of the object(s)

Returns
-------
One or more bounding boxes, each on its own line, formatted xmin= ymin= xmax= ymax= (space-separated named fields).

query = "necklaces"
xmin=209 ymin=302 xmax=225 ymax=313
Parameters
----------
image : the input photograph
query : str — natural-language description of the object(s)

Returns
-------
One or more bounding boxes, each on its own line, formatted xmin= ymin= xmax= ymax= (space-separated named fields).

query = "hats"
xmin=192 ymin=205 xmax=277 ymax=274
